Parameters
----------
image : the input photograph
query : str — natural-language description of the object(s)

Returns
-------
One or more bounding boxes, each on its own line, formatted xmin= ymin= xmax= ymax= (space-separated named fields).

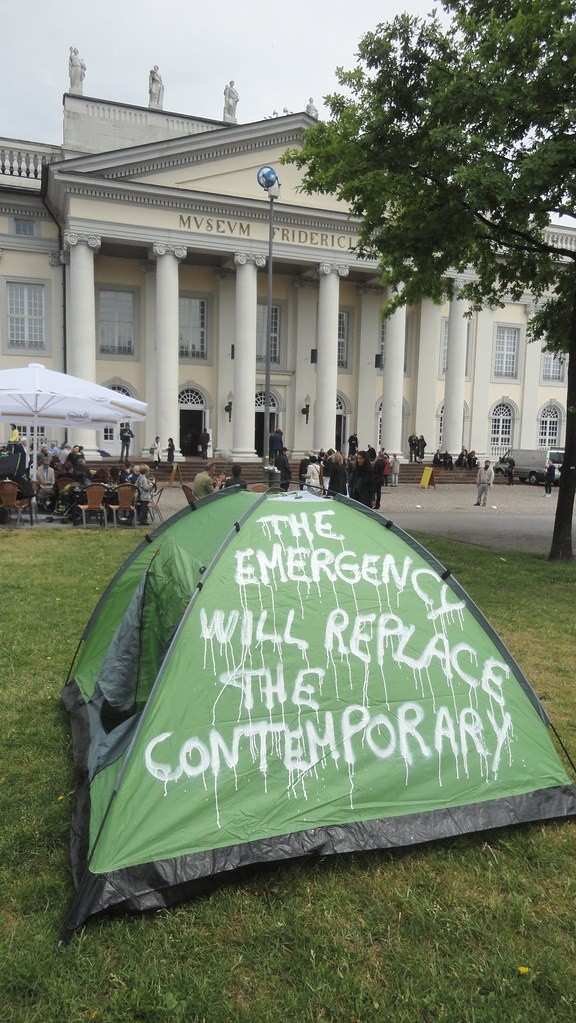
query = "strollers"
xmin=45 ymin=472 xmax=90 ymax=524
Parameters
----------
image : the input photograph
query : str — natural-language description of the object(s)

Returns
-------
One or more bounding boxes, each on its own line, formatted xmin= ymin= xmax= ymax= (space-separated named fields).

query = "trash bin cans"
xmin=269 ymin=468 xmax=282 ymax=492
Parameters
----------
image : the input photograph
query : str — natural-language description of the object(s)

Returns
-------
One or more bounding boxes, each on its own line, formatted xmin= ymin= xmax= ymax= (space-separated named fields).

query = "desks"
xmin=72 ymin=487 xmax=117 ymax=526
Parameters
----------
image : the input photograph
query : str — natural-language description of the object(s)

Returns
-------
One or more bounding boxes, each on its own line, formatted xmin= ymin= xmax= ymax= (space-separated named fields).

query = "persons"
xmin=505 ymin=456 xmax=515 ymax=486
xmin=147 ymin=65 xmax=165 ymax=111
xmin=68 ymin=46 xmax=86 ymax=95
xmin=223 ymin=80 xmax=240 ymax=124
xmin=473 ymin=460 xmax=494 ymax=506
xmin=305 ymin=97 xmax=319 ymax=120
xmin=541 ymin=458 xmax=556 ymax=498
xmin=1 ymin=423 xmax=480 ymax=525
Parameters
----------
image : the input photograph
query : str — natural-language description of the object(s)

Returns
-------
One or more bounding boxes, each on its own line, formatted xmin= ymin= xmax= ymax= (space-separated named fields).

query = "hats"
xmin=393 ymin=452 xmax=397 ymax=456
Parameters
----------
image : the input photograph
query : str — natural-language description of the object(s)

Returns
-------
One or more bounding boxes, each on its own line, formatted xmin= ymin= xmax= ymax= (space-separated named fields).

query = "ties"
xmin=44 ymin=469 xmax=47 ymax=480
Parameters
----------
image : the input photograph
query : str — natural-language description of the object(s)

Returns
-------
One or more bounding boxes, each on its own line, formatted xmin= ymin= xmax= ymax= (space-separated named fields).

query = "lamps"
xmin=302 ymin=393 xmax=310 ymax=424
xmin=225 ymin=389 xmax=233 ymax=422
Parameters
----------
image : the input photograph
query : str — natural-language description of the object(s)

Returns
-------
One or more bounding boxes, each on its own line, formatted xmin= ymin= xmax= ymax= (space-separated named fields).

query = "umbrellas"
xmin=1 ymin=363 xmax=148 ymax=522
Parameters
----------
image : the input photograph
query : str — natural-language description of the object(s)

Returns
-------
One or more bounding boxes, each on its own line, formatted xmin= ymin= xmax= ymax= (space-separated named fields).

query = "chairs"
xmin=0 ymin=478 xmax=286 ymax=529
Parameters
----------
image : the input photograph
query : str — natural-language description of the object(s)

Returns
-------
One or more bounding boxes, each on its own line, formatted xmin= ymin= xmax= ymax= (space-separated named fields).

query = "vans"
xmin=493 ymin=448 xmax=566 ymax=485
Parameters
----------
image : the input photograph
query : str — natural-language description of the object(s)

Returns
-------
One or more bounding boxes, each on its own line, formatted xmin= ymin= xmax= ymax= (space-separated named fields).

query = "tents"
xmin=53 ymin=481 xmax=575 ymax=949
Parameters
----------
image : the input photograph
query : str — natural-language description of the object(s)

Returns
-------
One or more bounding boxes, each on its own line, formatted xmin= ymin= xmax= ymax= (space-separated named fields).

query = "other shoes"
xmin=143 ymin=521 xmax=150 ymax=525
xmin=137 ymin=522 xmax=142 ymax=525
xmin=373 ymin=505 xmax=379 ymax=509
xmin=545 ymin=494 xmax=552 ymax=497
xmin=482 ymin=503 xmax=486 ymax=506
xmin=542 ymin=493 xmax=547 ymax=497
xmin=473 ymin=502 xmax=480 ymax=506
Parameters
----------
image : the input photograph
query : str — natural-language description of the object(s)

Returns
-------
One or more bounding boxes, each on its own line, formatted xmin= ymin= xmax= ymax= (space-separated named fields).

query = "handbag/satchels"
xmin=198 ymin=445 xmax=202 ymax=452
xmin=313 ymin=464 xmax=324 ymax=485
xmin=149 ymin=448 xmax=154 ymax=455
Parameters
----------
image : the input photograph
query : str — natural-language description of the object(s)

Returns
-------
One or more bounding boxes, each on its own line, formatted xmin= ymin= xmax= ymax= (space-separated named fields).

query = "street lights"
xmin=257 ymin=164 xmax=281 ymax=494
